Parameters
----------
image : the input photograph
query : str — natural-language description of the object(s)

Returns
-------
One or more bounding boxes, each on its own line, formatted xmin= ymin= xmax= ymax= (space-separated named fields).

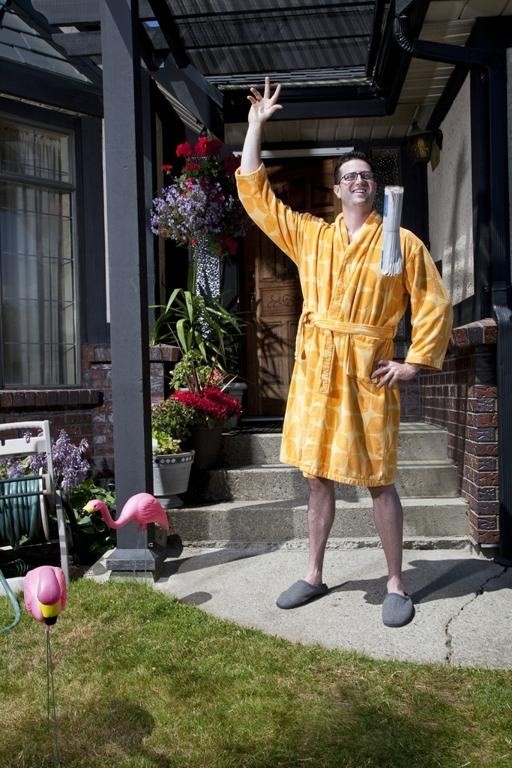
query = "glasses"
xmin=338 ymin=171 xmax=376 ymax=184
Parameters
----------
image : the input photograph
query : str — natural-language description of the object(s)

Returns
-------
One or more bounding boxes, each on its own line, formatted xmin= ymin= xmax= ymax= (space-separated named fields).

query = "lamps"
xmin=405 ymin=117 xmax=443 ymax=168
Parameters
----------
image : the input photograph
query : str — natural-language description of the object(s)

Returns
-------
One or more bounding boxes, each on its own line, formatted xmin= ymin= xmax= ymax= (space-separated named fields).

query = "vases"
xmin=184 ymin=427 xmax=225 ymax=470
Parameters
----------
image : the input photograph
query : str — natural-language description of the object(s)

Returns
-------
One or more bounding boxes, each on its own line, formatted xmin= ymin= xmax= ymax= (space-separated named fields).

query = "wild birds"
xmin=24 ymin=566 xmax=66 ymax=732
xmin=80 ymin=492 xmax=169 ymax=587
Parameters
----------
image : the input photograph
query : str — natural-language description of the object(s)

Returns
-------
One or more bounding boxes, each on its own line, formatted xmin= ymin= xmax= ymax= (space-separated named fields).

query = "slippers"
xmin=276 ymin=579 xmax=329 ymax=608
xmin=381 ymin=591 xmax=415 ymax=627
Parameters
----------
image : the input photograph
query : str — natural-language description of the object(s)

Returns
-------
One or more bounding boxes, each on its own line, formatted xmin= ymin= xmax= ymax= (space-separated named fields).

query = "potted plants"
xmin=151 ymin=398 xmax=197 ymax=496
xmin=152 ymin=281 xmax=273 ymax=429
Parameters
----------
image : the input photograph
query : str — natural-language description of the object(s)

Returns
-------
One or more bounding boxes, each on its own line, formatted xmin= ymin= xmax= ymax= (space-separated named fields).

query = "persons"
xmin=234 ymin=78 xmax=454 ymax=628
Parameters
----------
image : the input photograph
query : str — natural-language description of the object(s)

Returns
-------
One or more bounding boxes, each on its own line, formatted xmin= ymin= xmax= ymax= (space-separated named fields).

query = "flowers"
xmin=165 ymin=349 xmax=243 ymax=427
